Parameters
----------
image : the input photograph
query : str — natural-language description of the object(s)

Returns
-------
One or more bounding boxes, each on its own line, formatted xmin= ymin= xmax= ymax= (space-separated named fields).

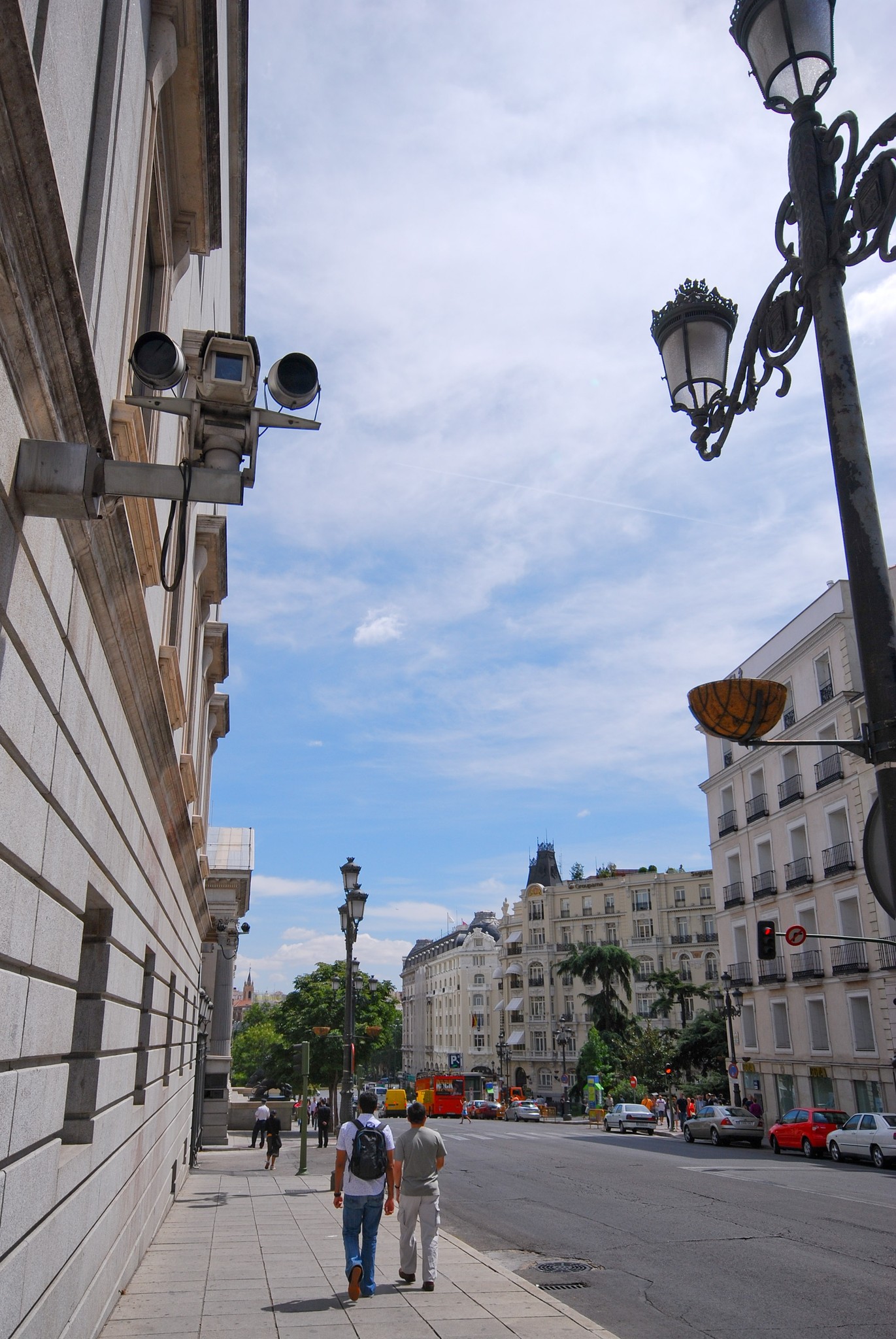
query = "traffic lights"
xmin=665 ymin=1062 xmax=672 ymax=1081
xmin=756 ymin=919 xmax=776 ymax=963
xmin=293 ymin=1042 xmax=308 ymax=1075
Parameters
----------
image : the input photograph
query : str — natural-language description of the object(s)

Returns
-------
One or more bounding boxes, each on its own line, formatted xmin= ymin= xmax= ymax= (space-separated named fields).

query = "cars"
xmin=768 ymin=1107 xmax=851 ymax=1159
xmin=682 ymin=1105 xmax=765 ymax=1149
xmin=603 ymin=1103 xmax=657 ymax=1135
xmin=825 ymin=1112 xmax=895 ymax=1170
xmin=505 ymin=1100 xmax=541 ymax=1122
xmin=474 ymin=1101 xmax=502 ymax=1120
xmin=466 ymin=1100 xmax=487 ymax=1119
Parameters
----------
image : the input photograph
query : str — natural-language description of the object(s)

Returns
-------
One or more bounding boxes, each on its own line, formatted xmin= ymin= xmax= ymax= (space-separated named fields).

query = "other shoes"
xmin=423 ymin=1281 xmax=435 ymax=1291
xmin=248 ymin=1145 xmax=255 ymax=1148
xmin=362 ymin=1293 xmax=375 ymax=1297
xmin=348 ymin=1267 xmax=361 ymax=1301
xmin=469 ymin=1121 xmax=471 ymax=1124
xmin=260 ymin=1146 xmax=262 ymax=1148
xmin=265 ymin=1161 xmax=269 ymax=1169
xmin=399 ymin=1267 xmax=416 ymax=1283
xmin=270 ymin=1166 xmax=276 ymax=1170
xmin=459 ymin=1123 xmax=463 ymax=1124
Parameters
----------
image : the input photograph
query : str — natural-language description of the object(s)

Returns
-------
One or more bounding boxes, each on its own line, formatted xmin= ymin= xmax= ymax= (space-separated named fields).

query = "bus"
xmin=415 ymin=1067 xmax=465 ymax=1119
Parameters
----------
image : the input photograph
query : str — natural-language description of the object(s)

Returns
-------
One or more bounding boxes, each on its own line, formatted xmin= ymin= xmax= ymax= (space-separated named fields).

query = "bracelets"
xmin=395 ymin=1185 xmax=400 ymax=1189
xmin=334 ymin=1192 xmax=342 ymax=1197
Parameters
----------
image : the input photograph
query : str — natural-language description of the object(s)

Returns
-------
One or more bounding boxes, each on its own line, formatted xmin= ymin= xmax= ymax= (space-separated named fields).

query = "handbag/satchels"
xmin=314 ymin=1108 xmax=318 ymax=1118
xmin=272 ymin=1134 xmax=282 ymax=1149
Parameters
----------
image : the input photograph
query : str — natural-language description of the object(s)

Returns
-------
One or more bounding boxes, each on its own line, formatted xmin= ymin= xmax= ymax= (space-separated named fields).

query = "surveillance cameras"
xmin=216 ymin=921 xmax=251 ymax=932
xmin=131 ymin=331 xmax=320 ymax=409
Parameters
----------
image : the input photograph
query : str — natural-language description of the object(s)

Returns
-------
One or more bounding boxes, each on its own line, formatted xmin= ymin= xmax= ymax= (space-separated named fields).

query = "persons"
xmin=247 ymin=1096 xmax=472 ymax=1170
xmin=391 ymin=1102 xmax=449 ymax=1291
xmin=641 ymin=1092 xmax=765 ymax=1131
xmin=333 ymin=1092 xmax=395 ymax=1301
xmin=495 ymin=1093 xmax=625 ymax=1120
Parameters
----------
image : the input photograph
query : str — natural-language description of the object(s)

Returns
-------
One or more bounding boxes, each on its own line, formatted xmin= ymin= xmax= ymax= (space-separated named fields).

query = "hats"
xmin=261 ymin=1098 xmax=267 ymax=1102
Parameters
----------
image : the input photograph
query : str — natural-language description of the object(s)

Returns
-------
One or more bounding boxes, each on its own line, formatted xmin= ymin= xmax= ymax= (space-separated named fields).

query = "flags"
xmin=448 ymin=914 xmax=467 ymax=925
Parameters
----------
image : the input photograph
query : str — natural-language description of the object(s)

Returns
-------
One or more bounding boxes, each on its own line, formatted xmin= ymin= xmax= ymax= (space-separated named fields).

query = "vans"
xmin=369 ymin=1086 xmax=388 ymax=1108
xmin=378 ymin=1078 xmax=389 ymax=1084
xmin=407 ymin=1103 xmax=412 ymax=1116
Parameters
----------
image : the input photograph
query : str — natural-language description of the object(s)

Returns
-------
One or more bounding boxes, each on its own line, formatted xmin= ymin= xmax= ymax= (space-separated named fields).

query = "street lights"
xmin=553 ymin=1016 xmax=573 ymax=1123
xmin=652 ymin=1 xmax=894 ymax=912
xmin=713 ymin=970 xmax=744 ymax=1108
xmin=337 ymin=856 xmax=370 ymax=1127
xmin=331 ymin=957 xmax=379 ymax=1084
xmin=487 ymin=1033 xmax=513 ymax=1103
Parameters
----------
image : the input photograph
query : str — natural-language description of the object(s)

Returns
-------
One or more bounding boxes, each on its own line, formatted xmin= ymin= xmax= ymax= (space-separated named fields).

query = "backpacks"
xmin=349 ymin=1118 xmax=387 ymax=1180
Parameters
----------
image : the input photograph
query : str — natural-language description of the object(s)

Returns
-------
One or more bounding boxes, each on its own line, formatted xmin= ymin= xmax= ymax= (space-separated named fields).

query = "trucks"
xmin=382 ymin=1089 xmax=407 ymax=1119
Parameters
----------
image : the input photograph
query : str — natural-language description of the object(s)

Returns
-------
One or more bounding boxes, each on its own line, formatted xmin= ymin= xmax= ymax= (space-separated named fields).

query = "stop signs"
xmin=629 ymin=1076 xmax=638 ymax=1089
